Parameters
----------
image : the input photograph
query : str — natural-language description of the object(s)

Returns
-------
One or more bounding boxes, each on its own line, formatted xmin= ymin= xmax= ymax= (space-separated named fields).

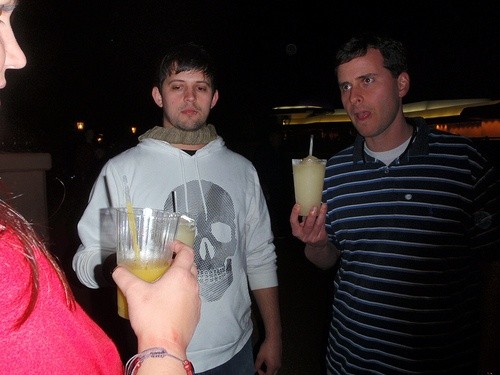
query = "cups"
xmin=176 ymin=214 xmax=196 ymax=248
xmin=101 ymin=208 xmax=117 ymax=266
xmin=118 ymin=208 xmax=178 ymax=318
xmin=292 ymin=159 xmax=327 ymax=217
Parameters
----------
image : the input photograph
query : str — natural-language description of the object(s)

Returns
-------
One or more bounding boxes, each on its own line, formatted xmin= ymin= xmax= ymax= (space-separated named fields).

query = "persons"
xmin=0 ymin=0 xmax=201 ymax=375
xmin=290 ymin=35 xmax=500 ymax=375
xmin=71 ymin=47 xmax=282 ymax=375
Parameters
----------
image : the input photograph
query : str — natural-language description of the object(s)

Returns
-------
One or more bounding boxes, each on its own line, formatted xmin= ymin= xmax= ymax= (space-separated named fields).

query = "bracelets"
xmin=123 ymin=348 xmax=195 ymax=374
